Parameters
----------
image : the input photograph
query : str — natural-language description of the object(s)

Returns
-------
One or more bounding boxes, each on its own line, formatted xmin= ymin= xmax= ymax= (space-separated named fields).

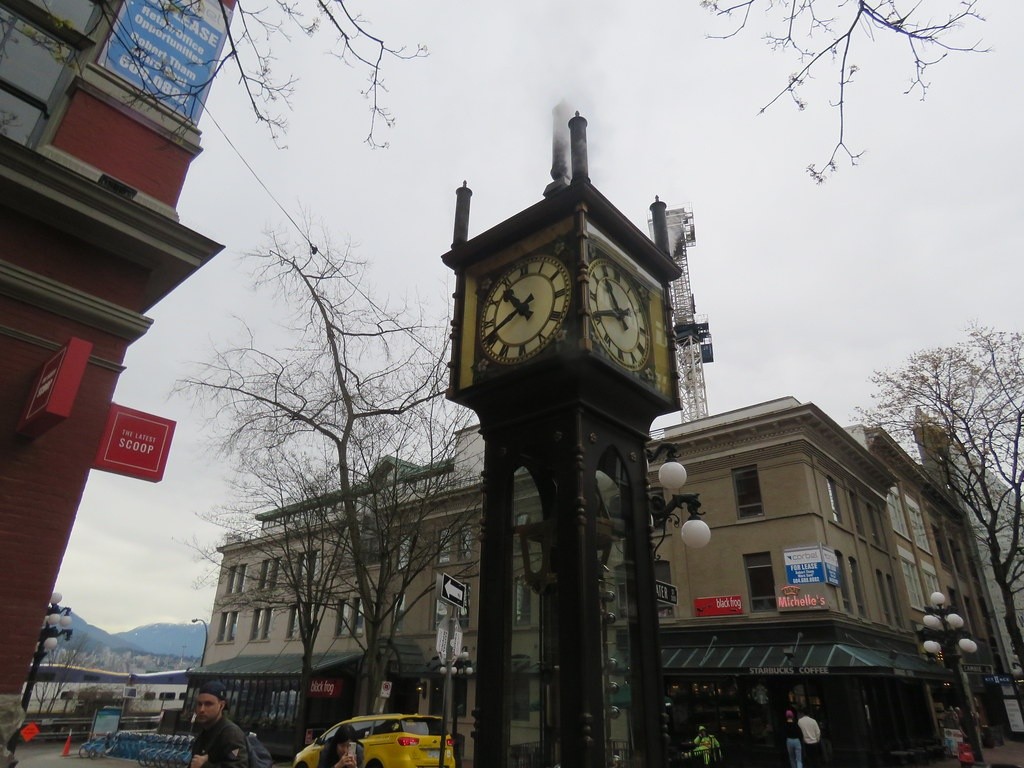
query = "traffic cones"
xmin=59 ymin=736 xmax=71 ymax=756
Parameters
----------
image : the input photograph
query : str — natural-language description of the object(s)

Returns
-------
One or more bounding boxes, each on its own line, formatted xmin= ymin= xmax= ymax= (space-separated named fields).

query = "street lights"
xmin=7 ymin=592 xmax=73 ymax=768
xmin=923 ymin=591 xmax=989 ymax=768
xmin=189 ymin=619 xmax=208 ymax=711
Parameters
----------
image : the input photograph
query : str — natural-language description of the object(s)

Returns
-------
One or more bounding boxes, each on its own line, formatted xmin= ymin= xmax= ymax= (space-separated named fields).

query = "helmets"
xmin=699 ymin=726 xmax=705 ymax=731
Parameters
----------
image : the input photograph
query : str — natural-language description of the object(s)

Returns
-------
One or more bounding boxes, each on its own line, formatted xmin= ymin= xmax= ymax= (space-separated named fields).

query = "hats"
xmin=199 ymin=680 xmax=229 ymax=710
xmin=786 ymin=711 xmax=793 ymax=718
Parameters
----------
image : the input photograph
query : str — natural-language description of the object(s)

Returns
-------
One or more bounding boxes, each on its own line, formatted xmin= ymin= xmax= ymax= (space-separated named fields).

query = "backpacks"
xmin=203 ymin=724 xmax=273 ymax=768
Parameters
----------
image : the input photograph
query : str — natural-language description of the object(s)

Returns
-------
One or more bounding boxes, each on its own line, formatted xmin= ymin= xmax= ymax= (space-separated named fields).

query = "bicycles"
xmin=137 ymin=733 xmax=196 ymax=768
xmin=79 ymin=731 xmax=125 ymax=760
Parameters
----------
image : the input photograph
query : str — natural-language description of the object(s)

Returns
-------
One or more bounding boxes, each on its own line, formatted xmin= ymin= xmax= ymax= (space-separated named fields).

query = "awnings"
xmin=660 ymin=644 xmax=958 ymax=683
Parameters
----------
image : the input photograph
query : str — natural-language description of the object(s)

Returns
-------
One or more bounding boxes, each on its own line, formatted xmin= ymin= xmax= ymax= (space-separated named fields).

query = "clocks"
xmin=583 ymin=259 xmax=654 ymax=372
xmin=479 ymin=254 xmax=575 ymax=364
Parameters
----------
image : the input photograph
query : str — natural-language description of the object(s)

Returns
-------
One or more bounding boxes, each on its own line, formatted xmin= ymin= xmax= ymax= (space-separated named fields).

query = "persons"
xmin=316 ymin=724 xmax=365 ymax=768
xmin=784 ymin=707 xmax=827 ymax=768
xmin=187 ymin=680 xmax=252 ymax=768
xmin=694 ymin=725 xmax=721 ymax=765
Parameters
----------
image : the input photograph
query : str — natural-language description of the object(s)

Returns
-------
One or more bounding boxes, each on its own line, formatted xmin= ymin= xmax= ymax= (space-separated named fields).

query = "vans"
xmin=293 ymin=714 xmax=455 ymax=768
